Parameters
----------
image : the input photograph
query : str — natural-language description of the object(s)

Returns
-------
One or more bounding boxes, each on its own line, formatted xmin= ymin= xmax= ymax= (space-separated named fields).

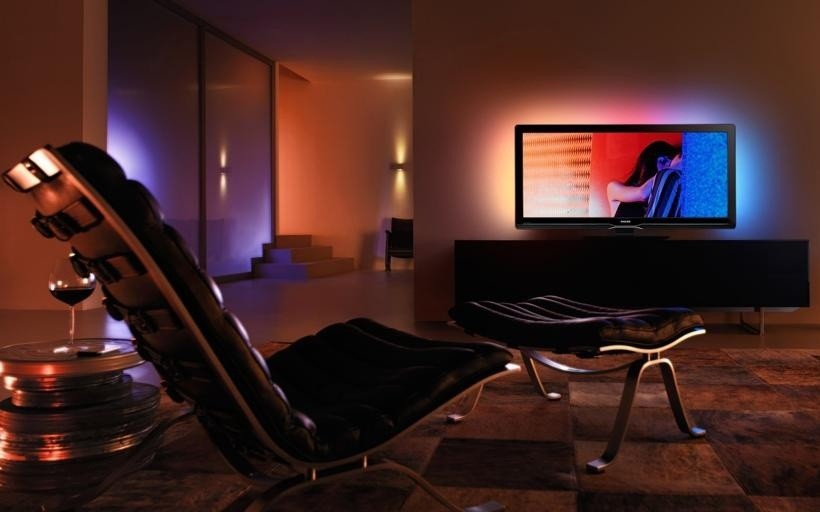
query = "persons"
xmin=646 ymin=144 xmax=681 ymax=219
xmin=606 ymin=140 xmax=682 ymax=218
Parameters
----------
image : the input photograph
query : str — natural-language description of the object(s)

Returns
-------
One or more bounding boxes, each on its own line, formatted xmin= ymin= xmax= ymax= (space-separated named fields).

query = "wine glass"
xmin=48 ymin=259 xmax=96 ymax=354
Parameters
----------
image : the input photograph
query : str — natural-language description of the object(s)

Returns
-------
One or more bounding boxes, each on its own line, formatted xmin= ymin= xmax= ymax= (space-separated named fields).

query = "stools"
xmin=457 ymin=293 xmax=707 ymax=474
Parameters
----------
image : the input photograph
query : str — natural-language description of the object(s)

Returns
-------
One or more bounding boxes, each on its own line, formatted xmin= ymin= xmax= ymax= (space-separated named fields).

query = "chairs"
xmin=2 ymin=140 xmax=522 ymax=511
